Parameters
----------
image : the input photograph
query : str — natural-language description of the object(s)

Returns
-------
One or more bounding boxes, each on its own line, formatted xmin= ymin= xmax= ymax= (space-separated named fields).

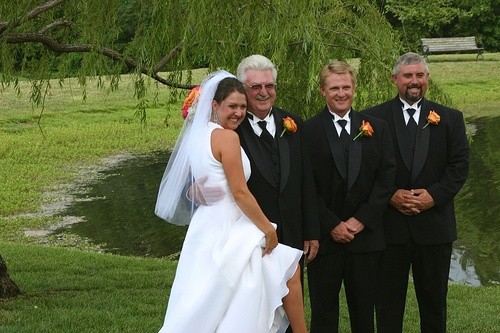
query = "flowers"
xmin=352 ymin=120 xmax=373 ymax=141
xmin=181 ymin=87 xmax=203 ymax=119
xmin=279 ymin=115 xmax=298 ymax=136
xmin=423 ymin=111 xmax=441 ymax=129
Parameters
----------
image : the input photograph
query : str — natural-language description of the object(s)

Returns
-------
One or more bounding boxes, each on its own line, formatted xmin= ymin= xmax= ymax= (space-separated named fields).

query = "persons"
xmin=154 ymin=70 xmax=307 ymax=333
xmin=360 ymin=51 xmax=470 ymax=333
xmin=181 ymin=56 xmax=322 ymax=333
xmin=305 ymin=59 xmax=396 ymax=333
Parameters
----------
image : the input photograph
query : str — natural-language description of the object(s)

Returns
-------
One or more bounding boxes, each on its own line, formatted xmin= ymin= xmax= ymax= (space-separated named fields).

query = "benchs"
xmin=421 ymin=36 xmax=484 ymax=63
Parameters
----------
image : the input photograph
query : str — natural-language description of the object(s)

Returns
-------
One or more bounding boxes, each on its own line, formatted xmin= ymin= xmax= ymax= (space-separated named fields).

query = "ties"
xmin=329 ymin=111 xmax=351 ymax=170
xmin=247 ymin=109 xmax=273 ymax=147
xmin=400 ymin=100 xmax=422 ymax=174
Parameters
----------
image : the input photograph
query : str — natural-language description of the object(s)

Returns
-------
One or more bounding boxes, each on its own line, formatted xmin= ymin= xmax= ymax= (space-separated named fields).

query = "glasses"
xmin=245 ymin=83 xmax=274 ymax=91
